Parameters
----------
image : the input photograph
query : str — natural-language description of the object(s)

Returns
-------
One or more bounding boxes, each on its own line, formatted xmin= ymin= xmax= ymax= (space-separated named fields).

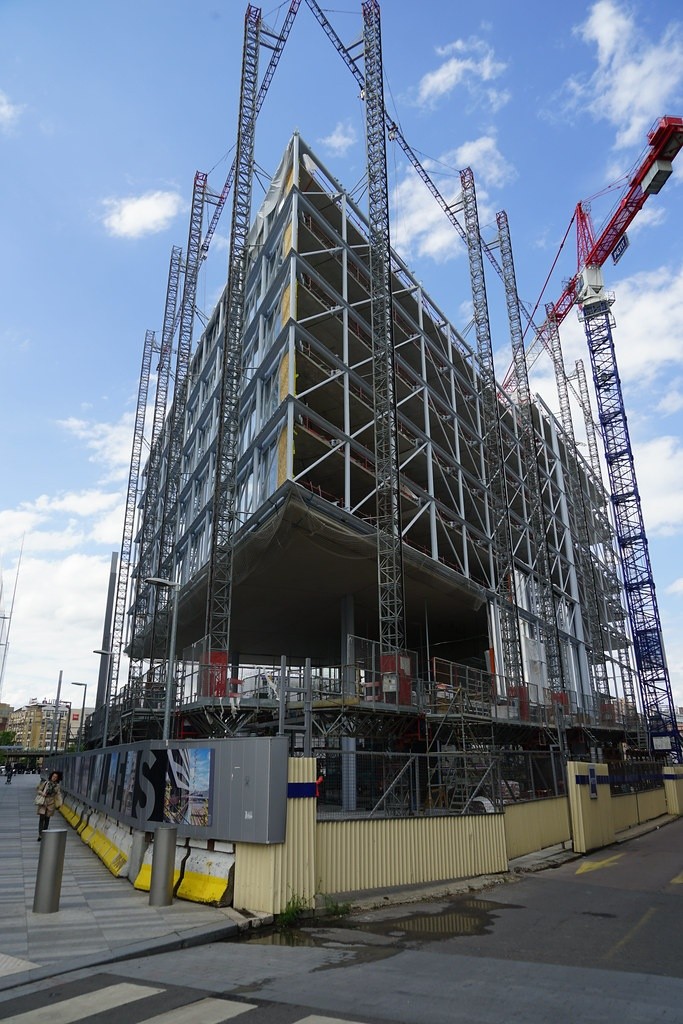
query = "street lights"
xmin=93 ymin=649 xmax=116 ymax=748
xmin=71 ymin=681 xmax=88 ymax=752
xmin=145 ymin=577 xmax=180 ymax=739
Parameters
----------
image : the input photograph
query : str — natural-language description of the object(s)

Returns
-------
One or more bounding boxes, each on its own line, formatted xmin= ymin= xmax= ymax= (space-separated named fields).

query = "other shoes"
xmin=37 ymin=836 xmax=42 ymax=842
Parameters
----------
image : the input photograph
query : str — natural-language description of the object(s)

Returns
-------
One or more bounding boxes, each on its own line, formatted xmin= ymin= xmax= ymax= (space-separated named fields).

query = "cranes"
xmin=494 ymin=114 xmax=683 ymax=766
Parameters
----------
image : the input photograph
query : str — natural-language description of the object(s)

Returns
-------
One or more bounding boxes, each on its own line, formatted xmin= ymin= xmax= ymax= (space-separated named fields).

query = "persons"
xmin=37 ymin=771 xmax=63 ymax=842
xmin=5 ymin=761 xmax=41 ymax=784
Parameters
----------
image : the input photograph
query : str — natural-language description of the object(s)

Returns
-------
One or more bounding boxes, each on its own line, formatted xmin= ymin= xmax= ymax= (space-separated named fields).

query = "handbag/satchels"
xmin=34 ymin=781 xmax=49 ymax=805
